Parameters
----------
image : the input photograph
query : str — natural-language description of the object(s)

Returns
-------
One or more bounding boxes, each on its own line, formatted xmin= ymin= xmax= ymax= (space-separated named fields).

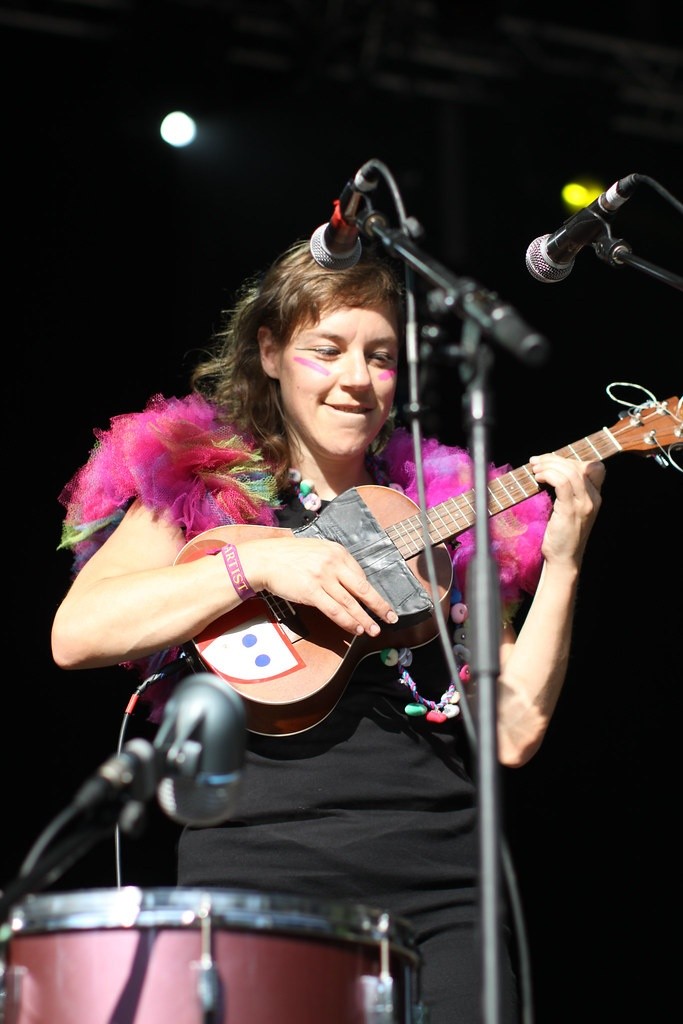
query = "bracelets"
xmin=205 ymin=544 xmax=258 ymax=603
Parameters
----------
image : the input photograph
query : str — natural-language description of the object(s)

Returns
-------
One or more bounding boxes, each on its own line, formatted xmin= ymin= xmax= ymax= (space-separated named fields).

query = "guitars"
xmin=178 ymin=381 xmax=683 ymax=736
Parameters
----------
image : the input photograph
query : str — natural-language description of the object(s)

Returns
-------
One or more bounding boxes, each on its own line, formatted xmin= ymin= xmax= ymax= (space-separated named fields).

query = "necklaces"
xmin=286 ymin=463 xmax=406 ymax=510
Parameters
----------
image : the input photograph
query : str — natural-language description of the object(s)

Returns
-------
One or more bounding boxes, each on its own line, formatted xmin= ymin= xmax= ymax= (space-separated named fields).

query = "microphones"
xmin=526 ymin=175 xmax=640 ymax=284
xmin=310 ymin=160 xmax=378 ymax=269
xmin=31 ymin=674 xmax=243 ymax=888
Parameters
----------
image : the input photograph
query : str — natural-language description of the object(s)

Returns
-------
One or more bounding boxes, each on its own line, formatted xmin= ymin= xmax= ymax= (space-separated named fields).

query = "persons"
xmin=48 ymin=235 xmax=609 ymax=1023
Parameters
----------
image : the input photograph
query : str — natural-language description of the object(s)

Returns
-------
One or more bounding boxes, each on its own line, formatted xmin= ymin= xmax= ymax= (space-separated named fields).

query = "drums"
xmin=0 ymin=886 xmax=423 ymax=1024
